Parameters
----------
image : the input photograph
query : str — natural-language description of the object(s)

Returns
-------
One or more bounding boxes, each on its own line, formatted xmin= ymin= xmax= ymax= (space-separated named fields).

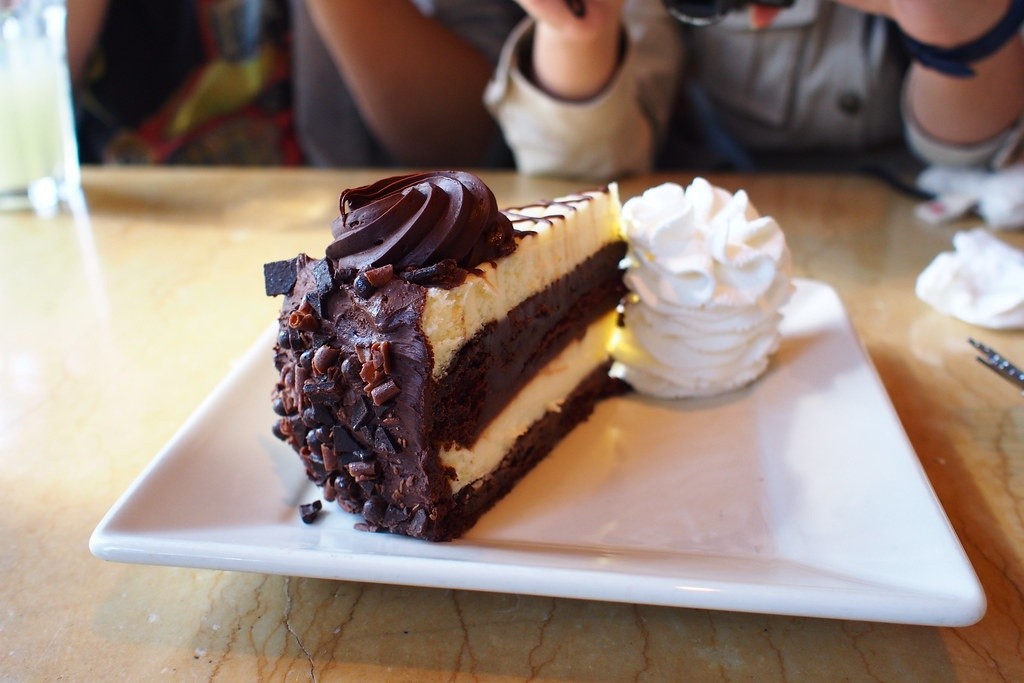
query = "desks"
xmin=0 ymin=169 xmax=1024 ymax=683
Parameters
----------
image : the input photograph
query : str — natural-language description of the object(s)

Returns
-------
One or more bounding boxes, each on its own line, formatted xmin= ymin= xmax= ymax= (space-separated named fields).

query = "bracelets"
xmin=894 ymin=0 xmax=1024 ymax=79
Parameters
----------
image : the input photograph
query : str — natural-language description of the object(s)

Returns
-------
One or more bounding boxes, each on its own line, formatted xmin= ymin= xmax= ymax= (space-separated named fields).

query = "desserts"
xmin=261 ymin=169 xmax=798 ymax=539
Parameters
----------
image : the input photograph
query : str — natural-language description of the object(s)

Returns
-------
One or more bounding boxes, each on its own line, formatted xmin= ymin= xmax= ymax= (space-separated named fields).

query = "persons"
xmin=483 ymin=0 xmax=1024 ymax=182
xmin=65 ymin=1 xmax=495 ymax=175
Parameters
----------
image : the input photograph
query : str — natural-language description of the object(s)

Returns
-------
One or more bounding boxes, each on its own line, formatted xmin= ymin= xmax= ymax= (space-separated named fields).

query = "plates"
xmin=88 ymin=278 xmax=989 ymax=628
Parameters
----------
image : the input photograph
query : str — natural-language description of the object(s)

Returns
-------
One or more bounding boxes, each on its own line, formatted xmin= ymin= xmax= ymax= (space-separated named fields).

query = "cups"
xmin=2 ymin=0 xmax=82 ymax=208
